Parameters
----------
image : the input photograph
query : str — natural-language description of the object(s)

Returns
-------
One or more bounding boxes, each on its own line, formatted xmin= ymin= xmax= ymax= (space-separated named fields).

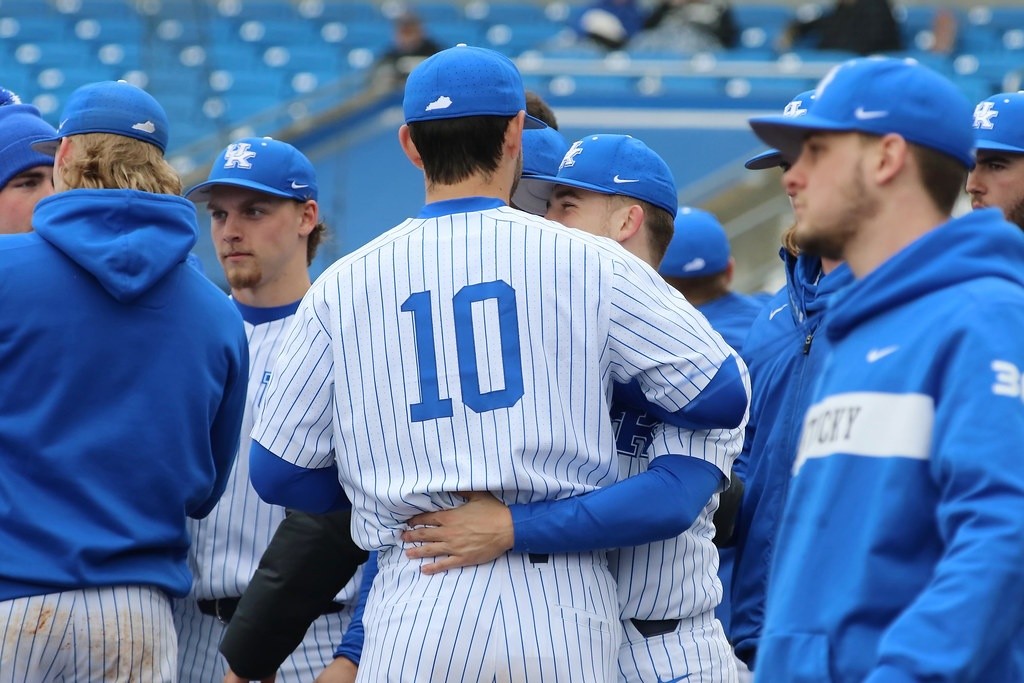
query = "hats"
xmin=521 ymin=124 xmax=569 ymax=176
xmin=747 ymin=54 xmax=978 ymax=171
xmin=510 ymin=133 xmax=678 ymax=225
xmin=29 ymin=79 xmax=168 ymax=157
xmin=973 ymin=90 xmax=1024 ymax=153
xmin=0 ymin=86 xmax=60 ymax=189
xmin=184 ymin=136 xmax=319 ymax=205
xmin=402 ymin=43 xmax=548 ymax=130
xmin=655 ymin=207 xmax=729 ymax=277
xmin=746 ymin=86 xmax=818 ymax=170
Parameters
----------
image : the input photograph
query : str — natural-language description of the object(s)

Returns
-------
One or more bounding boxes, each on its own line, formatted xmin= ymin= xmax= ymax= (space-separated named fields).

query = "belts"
xmin=198 ymin=596 xmax=344 ymax=624
xmin=629 ymin=612 xmax=676 ymax=637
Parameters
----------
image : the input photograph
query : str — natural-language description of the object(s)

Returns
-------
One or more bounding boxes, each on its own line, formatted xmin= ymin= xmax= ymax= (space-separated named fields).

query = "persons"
xmin=572 ymin=0 xmax=739 ymax=50
xmin=656 ymin=206 xmax=776 ymax=683
xmin=775 ymin=0 xmax=907 ymax=53
xmin=741 ymin=56 xmax=1024 ymax=683
xmin=180 ymin=136 xmax=380 ymax=683
xmin=0 ymin=80 xmax=247 ymax=683
xmin=246 ymin=45 xmax=747 ymax=683
xmin=404 ymin=135 xmax=752 ymax=683
xmin=373 ymin=15 xmax=436 ymax=85
xmin=0 ymin=89 xmax=57 ymax=234
